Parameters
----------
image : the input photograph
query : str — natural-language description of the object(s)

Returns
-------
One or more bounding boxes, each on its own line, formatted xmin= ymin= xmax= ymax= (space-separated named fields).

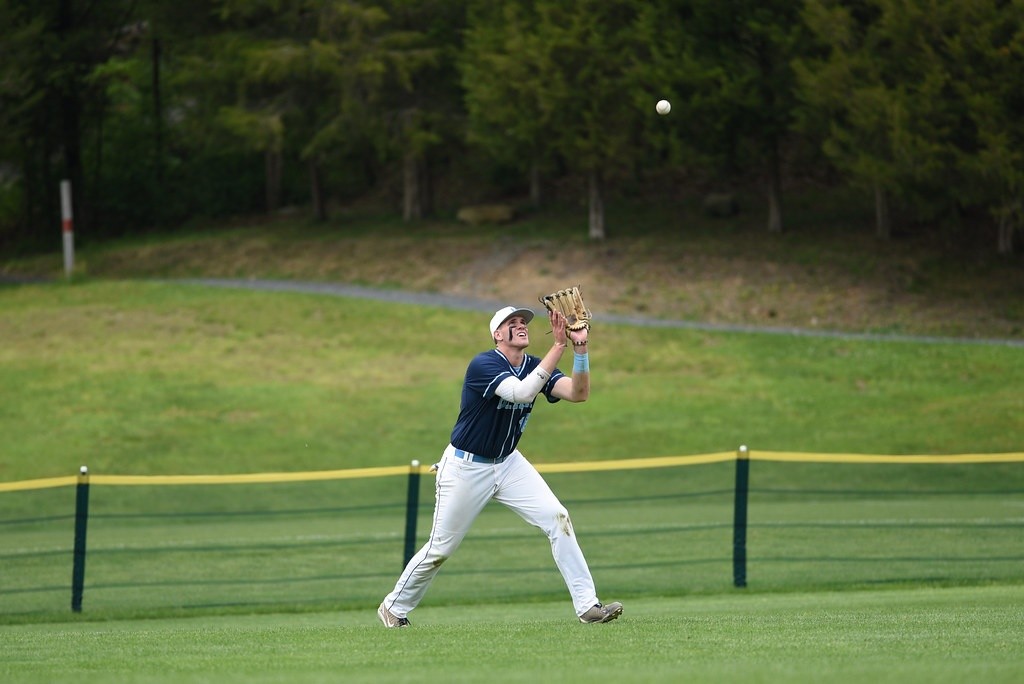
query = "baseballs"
xmin=655 ymin=99 xmax=671 ymax=116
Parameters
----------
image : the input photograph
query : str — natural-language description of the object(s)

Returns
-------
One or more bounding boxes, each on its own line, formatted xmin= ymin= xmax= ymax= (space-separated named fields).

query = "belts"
xmin=456 ymin=449 xmax=508 ymax=464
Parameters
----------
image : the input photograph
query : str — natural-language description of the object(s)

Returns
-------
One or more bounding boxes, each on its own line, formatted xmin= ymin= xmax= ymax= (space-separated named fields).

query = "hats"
xmin=490 ymin=305 xmax=535 ymax=344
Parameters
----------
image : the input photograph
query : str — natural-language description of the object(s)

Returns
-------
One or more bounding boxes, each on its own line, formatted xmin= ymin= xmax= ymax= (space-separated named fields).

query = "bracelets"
xmin=572 ymin=340 xmax=586 ymax=346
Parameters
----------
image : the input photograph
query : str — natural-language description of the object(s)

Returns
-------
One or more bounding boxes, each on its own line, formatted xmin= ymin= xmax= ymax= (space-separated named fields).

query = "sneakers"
xmin=579 ymin=601 xmax=623 ymax=625
xmin=378 ymin=602 xmax=411 ymax=629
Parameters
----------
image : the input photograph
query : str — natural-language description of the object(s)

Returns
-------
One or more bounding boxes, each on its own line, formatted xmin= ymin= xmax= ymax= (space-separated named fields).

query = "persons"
xmin=376 ymin=306 xmax=623 ymax=630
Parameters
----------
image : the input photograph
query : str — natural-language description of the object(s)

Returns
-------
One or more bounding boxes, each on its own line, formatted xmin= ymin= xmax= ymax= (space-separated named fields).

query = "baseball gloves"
xmin=543 ymin=287 xmax=591 ymax=337
